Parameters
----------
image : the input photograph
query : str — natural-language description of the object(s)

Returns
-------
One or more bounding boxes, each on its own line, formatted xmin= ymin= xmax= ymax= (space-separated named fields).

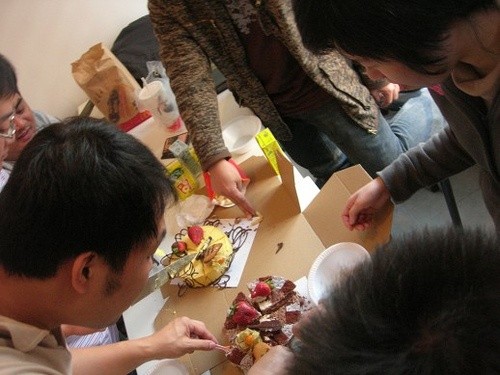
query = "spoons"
xmin=215 ymin=344 xmax=237 ymax=358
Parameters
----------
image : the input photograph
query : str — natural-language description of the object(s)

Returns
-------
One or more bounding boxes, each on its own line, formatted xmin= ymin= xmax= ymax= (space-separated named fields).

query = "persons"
xmin=146 ymin=0 xmax=450 ymax=221
xmin=0 ymin=115 xmax=219 ymax=375
xmin=246 ymin=224 xmax=500 ymax=375
xmin=0 ymin=52 xmax=138 ymax=375
xmin=290 ymin=0 xmax=500 ymax=240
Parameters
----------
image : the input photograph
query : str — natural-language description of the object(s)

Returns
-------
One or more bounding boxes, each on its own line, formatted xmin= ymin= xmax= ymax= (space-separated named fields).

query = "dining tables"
xmin=77 ymin=85 xmax=327 ymax=375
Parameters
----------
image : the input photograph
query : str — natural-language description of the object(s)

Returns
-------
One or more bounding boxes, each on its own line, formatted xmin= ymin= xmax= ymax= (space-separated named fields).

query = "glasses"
xmin=0 ymin=109 xmax=16 ymax=139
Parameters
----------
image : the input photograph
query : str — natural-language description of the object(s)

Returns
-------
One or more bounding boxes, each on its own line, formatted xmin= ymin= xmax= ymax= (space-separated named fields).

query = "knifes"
xmin=129 ymin=253 xmax=198 ymax=305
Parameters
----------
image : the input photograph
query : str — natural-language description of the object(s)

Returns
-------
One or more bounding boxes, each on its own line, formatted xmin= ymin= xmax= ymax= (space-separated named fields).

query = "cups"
xmin=138 ymin=80 xmax=182 ymax=132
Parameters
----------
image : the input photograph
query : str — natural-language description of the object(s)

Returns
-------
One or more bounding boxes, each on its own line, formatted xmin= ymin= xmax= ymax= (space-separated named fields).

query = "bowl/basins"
xmin=220 ymin=115 xmax=262 ymax=156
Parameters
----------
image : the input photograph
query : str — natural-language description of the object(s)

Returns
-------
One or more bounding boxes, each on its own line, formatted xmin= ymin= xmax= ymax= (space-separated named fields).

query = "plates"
xmin=306 ymin=242 xmax=373 ymax=307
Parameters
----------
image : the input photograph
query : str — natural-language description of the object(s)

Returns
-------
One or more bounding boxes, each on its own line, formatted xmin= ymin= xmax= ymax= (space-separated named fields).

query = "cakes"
xmin=166 ymin=225 xmax=233 ymax=289
xmin=222 ymin=275 xmax=312 ymax=372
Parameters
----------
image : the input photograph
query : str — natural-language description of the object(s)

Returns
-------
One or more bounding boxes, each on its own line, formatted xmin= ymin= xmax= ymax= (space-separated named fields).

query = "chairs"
xmin=385 ymin=87 xmax=463 ymax=229
